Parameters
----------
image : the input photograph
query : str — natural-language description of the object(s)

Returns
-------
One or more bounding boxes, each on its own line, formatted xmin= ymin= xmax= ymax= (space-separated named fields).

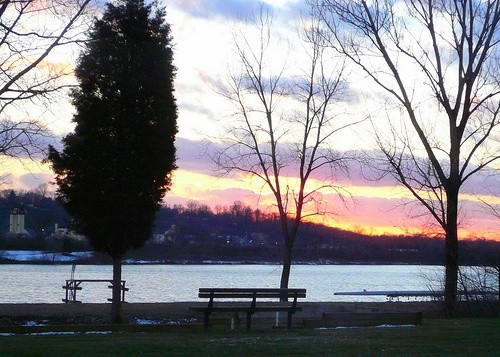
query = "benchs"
xmin=188 ymin=287 xmax=306 ymax=331
